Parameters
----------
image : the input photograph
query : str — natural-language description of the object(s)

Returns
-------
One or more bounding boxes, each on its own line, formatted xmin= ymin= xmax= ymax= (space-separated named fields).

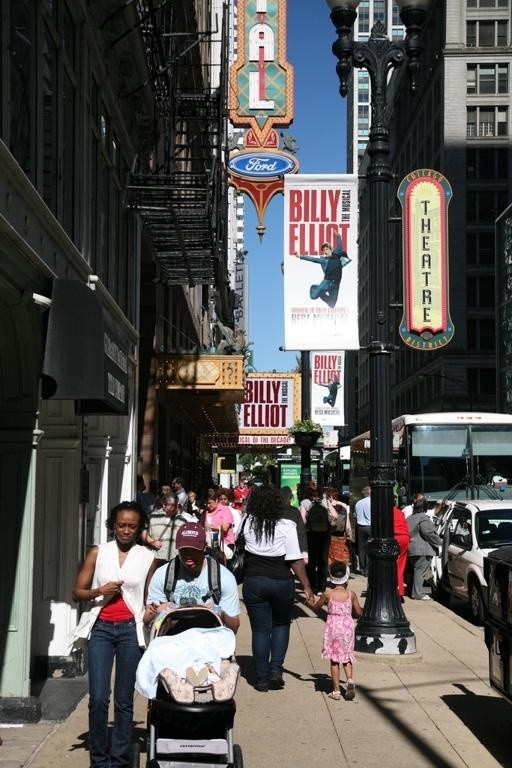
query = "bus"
xmin=324 ymin=445 xmax=350 ymax=506
xmin=349 ymin=411 xmax=511 ymax=518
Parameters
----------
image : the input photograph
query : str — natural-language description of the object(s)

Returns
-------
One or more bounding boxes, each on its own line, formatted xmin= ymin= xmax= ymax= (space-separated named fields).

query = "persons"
xmin=73 ymin=501 xmax=157 ymax=768
xmin=235 ymin=486 xmax=314 ymax=691
xmin=295 ymin=229 xmax=350 ymax=308
xmin=145 ymin=477 xmax=373 ymax=593
xmin=317 ymin=370 xmax=338 ymax=408
xmin=144 ymin=523 xmax=240 ymax=636
xmin=394 ymin=495 xmax=446 ymax=602
xmin=308 ymin=562 xmax=363 ymax=699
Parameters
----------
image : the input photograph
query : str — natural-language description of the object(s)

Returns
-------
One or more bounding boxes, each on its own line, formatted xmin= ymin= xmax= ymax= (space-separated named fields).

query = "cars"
xmin=482 ymin=545 xmax=511 ymax=705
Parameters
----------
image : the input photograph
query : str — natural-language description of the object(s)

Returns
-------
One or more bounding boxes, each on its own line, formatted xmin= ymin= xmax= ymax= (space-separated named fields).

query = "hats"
xmin=176 ymin=522 xmax=206 ymax=550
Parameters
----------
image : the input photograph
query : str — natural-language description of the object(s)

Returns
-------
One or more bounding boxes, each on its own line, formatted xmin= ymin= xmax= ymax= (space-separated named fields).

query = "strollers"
xmin=146 ymin=602 xmax=247 ymax=768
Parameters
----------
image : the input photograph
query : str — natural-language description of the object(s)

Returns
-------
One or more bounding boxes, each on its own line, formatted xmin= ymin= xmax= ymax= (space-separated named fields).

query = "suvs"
xmin=430 ymin=500 xmax=511 ymax=623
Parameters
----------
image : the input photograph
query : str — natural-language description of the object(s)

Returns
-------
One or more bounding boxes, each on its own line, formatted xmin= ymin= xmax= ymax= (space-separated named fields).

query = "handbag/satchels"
xmin=230 ymin=535 xmax=246 ymax=584
xmin=71 ymin=638 xmax=88 ymax=676
xmin=329 ymin=535 xmax=350 ymax=564
xmin=206 ymin=539 xmax=232 ymax=565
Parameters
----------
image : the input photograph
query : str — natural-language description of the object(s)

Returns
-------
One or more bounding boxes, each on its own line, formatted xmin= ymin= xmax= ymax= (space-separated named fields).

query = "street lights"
xmin=325 ymin=0 xmax=427 ymax=657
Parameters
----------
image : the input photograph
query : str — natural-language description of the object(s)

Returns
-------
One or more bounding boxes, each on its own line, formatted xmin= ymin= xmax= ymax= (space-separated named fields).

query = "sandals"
xmin=329 ymin=691 xmax=341 ymax=699
xmin=345 ymin=680 xmax=355 ymax=699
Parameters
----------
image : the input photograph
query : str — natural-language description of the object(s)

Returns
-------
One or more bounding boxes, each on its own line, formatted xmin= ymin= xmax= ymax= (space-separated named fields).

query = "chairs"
xmin=454 ymin=519 xmax=512 ymax=542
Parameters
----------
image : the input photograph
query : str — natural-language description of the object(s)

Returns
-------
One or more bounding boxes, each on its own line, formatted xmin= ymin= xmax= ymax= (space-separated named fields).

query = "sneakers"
xmin=269 ymin=672 xmax=284 ymax=686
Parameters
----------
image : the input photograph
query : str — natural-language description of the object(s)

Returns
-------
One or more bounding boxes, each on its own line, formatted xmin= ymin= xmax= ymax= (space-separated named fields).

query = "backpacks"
xmin=305 ymin=497 xmax=329 ymax=532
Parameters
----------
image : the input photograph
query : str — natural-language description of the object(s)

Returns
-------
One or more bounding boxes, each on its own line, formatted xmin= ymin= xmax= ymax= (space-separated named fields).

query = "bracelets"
xmin=88 ymin=589 xmax=99 ymax=600
